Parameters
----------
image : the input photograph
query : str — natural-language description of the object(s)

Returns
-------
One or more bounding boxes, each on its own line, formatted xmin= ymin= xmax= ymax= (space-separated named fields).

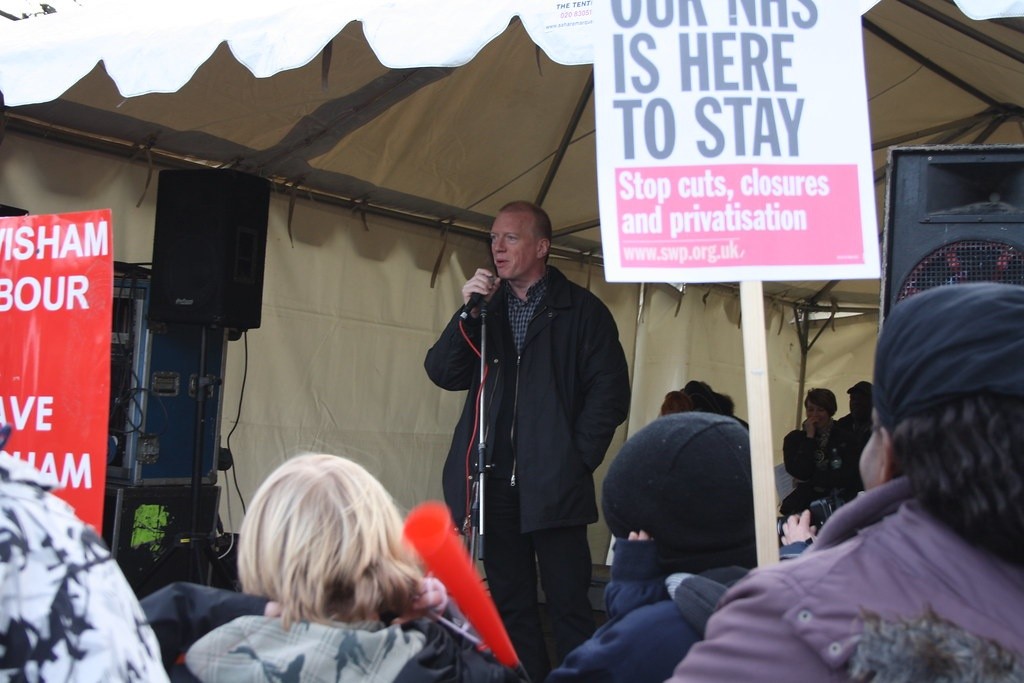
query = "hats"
xmin=872 ymin=283 xmax=1023 ymax=432
xmin=601 ymin=412 xmax=758 ymax=569
xmin=846 ymin=381 xmax=872 ymax=400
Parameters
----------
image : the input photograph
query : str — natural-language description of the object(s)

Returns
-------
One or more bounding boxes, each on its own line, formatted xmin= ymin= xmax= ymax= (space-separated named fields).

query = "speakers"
xmin=149 ymin=169 xmax=271 ymax=331
xmin=878 ymin=142 xmax=1024 ymax=336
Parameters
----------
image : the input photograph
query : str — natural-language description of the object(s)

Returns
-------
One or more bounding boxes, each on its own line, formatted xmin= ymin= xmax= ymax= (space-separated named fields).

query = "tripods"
xmin=135 ymin=327 xmax=235 ymax=600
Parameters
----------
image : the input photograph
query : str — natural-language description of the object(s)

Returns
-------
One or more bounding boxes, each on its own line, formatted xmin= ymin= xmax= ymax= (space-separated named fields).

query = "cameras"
xmin=777 ymin=488 xmax=853 ymax=538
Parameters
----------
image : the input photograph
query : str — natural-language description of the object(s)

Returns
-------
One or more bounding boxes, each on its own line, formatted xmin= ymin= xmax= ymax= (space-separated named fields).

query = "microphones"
xmin=460 ymin=273 xmax=497 ymax=320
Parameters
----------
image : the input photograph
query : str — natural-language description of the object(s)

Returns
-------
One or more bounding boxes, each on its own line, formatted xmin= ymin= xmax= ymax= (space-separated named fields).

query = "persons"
xmin=546 ymin=411 xmax=758 ymax=683
xmin=0 ymin=447 xmax=173 ymax=683
xmin=662 ymin=281 xmax=1024 ymax=683
xmin=424 ymin=200 xmax=632 ymax=683
xmin=139 ymin=452 xmax=524 ymax=683
xmin=658 ymin=374 xmax=875 ymax=560
